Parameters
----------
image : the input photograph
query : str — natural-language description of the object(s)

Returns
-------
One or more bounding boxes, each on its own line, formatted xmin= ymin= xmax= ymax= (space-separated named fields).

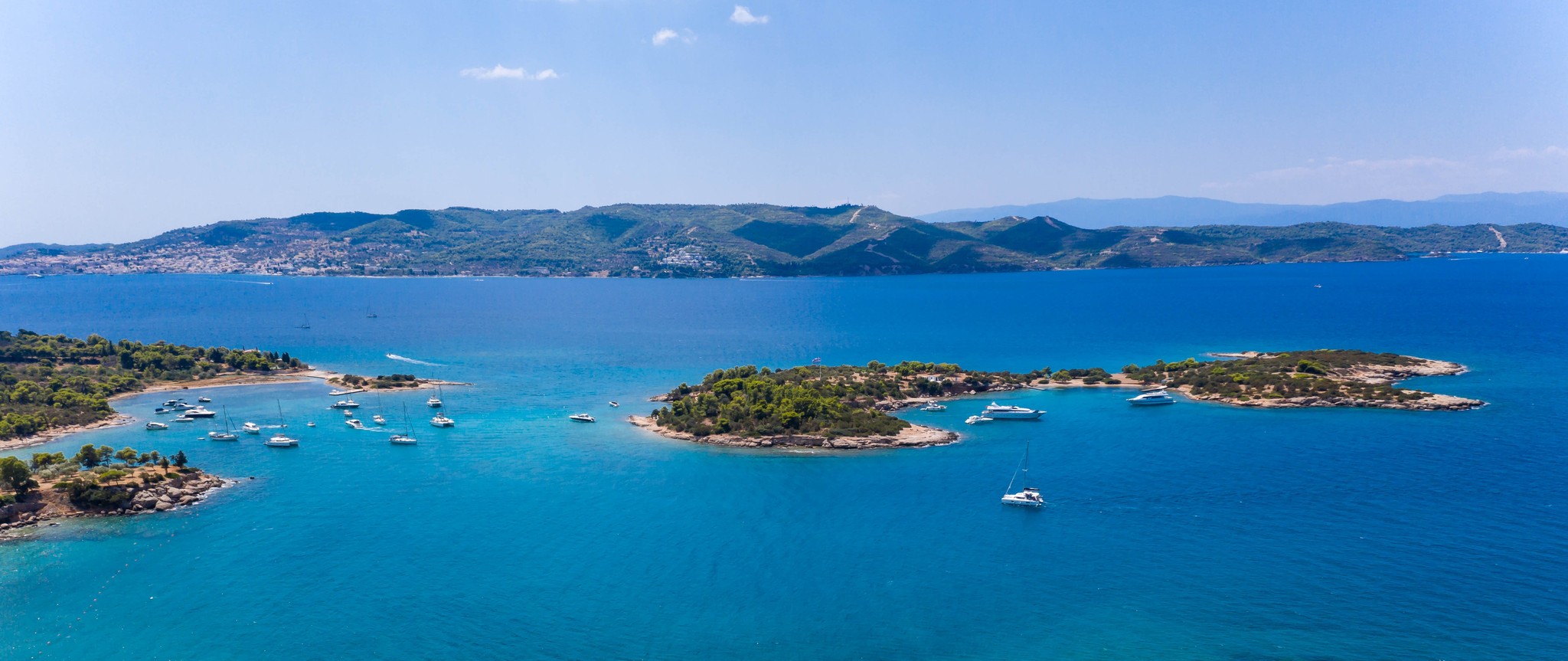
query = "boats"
xmin=163 ymin=398 xmax=217 ymax=418
xmin=568 ymin=413 xmax=596 ymax=422
xmin=146 ymin=422 xmax=169 ymax=430
xmin=344 ymin=410 xmax=353 ymax=417
xmin=980 ymin=402 xmax=1047 ymax=419
xmin=198 ymin=438 xmax=206 ymax=440
xmin=173 ymin=417 xmax=194 ymax=422
xmin=154 ymin=408 xmax=170 ymax=415
xmin=198 ymin=396 xmax=211 ymax=402
xmin=242 ymin=421 xmax=260 ymax=434
xmin=920 ymin=400 xmax=946 ymax=411
xmin=1125 ymin=391 xmax=1176 ymax=404
xmin=1141 ymin=384 xmax=1168 ymax=392
xmin=345 ymin=417 xmax=363 ymax=428
xmin=330 ymin=400 xmax=360 ymax=409
xmin=307 ymin=421 xmax=315 ymax=427
xmin=964 ymin=416 xmax=993 ymax=424
xmin=609 ymin=401 xmax=620 ymax=407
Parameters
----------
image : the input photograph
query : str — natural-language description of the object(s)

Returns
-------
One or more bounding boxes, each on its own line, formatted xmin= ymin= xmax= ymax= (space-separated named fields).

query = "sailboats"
xmin=388 ymin=400 xmax=418 ymax=444
xmin=365 ymin=302 xmax=377 ymax=317
xmin=300 ymin=314 xmax=311 ymax=328
xmin=427 ymin=377 xmax=455 ymax=427
xmin=426 ymin=371 xmax=442 ymax=407
xmin=262 ymin=399 xmax=298 ymax=447
xmin=344 ymin=383 xmax=355 ymax=403
xmin=372 ymin=387 xmax=387 ymax=426
xmin=211 ymin=404 xmax=241 ymax=440
xmin=999 ymin=438 xmax=1046 ymax=507
